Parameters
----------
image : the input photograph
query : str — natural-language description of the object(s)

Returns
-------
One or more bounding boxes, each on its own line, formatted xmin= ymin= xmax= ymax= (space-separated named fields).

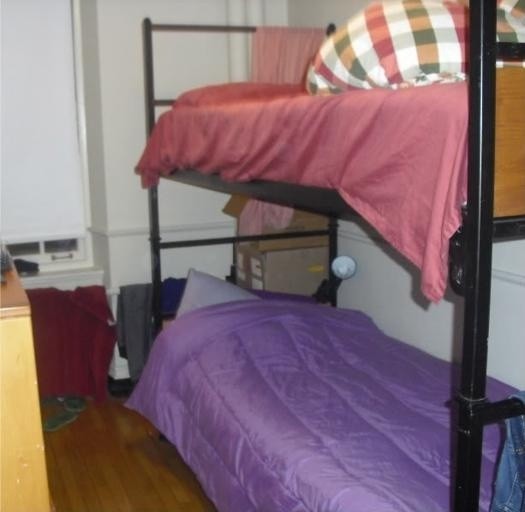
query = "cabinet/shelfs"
xmin=0 ymin=251 xmax=53 ymax=511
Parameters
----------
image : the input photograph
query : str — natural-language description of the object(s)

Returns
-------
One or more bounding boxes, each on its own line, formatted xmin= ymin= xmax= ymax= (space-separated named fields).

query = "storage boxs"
xmin=233 ymin=244 xmax=329 ymax=297
xmin=221 ymin=194 xmax=331 ymax=251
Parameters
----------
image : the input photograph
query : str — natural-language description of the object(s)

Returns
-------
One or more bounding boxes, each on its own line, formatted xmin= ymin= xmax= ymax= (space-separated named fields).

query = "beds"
xmin=137 ymin=0 xmax=524 ymax=512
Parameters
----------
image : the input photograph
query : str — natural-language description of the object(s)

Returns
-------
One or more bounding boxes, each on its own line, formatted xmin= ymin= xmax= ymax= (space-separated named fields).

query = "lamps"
xmin=327 ymin=253 xmax=358 ymax=308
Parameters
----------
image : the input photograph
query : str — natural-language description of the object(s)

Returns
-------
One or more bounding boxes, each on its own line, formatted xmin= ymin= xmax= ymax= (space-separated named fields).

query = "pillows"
xmin=175 ymin=79 xmax=306 ymax=107
xmin=173 ymin=269 xmax=262 ymax=321
